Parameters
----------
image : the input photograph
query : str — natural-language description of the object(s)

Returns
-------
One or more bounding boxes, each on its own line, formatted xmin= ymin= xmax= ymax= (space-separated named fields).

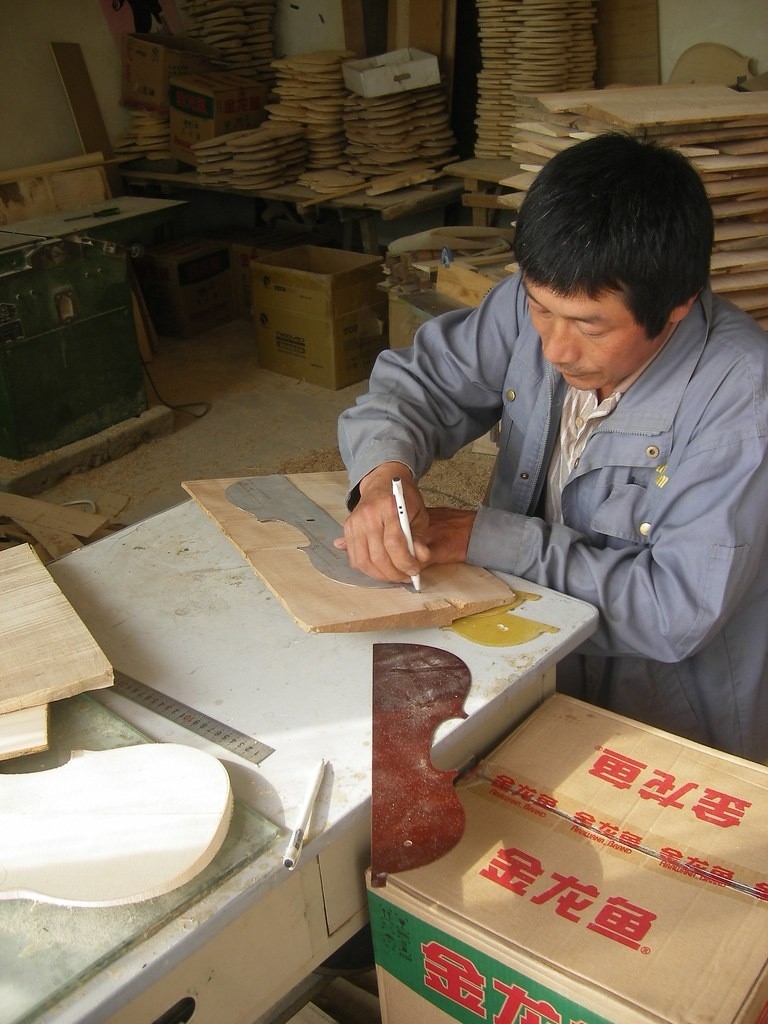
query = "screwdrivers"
xmin=63 ymin=207 xmax=121 ymax=222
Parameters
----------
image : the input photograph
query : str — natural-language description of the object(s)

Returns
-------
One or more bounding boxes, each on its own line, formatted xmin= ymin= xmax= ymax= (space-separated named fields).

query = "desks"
xmin=0 ymin=196 xmax=190 ymax=364
xmin=120 ymin=169 xmax=471 ymax=256
xmin=0 ymin=494 xmax=600 ymax=1024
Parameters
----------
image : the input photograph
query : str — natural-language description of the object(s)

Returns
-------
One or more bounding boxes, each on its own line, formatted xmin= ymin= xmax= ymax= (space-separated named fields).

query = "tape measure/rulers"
xmin=105 ymin=668 xmax=274 ymax=764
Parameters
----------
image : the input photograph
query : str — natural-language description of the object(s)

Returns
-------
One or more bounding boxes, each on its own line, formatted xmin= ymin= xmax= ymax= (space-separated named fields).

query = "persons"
xmin=333 ymin=129 xmax=768 ymax=767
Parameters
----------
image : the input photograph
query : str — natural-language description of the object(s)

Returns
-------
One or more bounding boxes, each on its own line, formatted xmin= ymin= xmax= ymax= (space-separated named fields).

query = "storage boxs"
xmin=117 ymin=31 xmax=221 ymax=115
xmin=124 ymin=236 xmax=240 ymax=338
xmin=364 ymin=693 xmax=768 ymax=1024
xmin=194 ymin=224 xmax=255 ymax=252
xmin=232 ymin=228 xmax=340 ymax=323
xmin=169 ymin=70 xmax=268 ymax=168
xmin=247 ymin=244 xmax=390 ymax=390
xmin=340 ymin=47 xmax=442 ymax=99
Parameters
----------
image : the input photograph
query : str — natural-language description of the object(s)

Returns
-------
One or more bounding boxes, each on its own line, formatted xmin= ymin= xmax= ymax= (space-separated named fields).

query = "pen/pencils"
xmin=390 ymin=476 xmax=425 ymax=594
xmin=283 ymin=757 xmax=326 ymax=873
